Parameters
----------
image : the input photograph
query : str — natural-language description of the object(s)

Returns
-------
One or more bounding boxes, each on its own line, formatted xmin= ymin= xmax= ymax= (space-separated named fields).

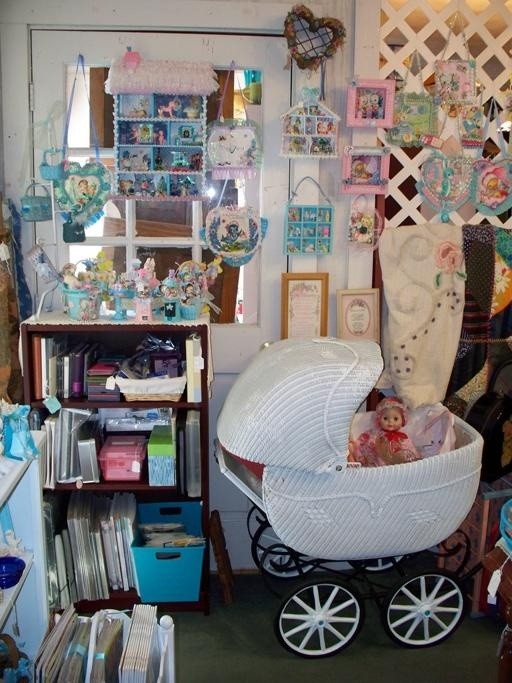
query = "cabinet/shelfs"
xmin=1 ymin=428 xmax=49 ymax=683
xmin=438 ymin=473 xmax=512 ymax=620
xmin=20 ymin=321 xmax=210 ymax=612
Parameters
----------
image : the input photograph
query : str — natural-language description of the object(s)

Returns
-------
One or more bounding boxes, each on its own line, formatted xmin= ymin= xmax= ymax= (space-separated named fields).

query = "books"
xmin=41 ymin=490 xmax=140 ymax=609
xmin=34 ymin=604 xmax=176 ymax=683
xmin=34 ymin=408 xmax=202 ymax=498
xmin=34 ymin=332 xmax=204 ymax=403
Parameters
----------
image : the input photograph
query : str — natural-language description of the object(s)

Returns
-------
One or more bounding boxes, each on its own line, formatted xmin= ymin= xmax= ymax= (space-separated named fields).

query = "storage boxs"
xmin=129 ymin=503 xmax=207 ymax=604
xmin=97 ymin=435 xmax=146 ymax=482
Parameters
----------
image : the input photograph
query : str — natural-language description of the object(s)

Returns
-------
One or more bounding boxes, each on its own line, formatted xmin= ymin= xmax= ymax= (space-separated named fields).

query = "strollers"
xmin=216 ymin=337 xmax=485 ymax=660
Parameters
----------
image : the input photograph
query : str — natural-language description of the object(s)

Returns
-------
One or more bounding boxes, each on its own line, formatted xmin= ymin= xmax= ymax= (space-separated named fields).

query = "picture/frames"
xmin=337 ymin=289 xmax=381 ymax=345
xmin=281 ymin=273 xmax=328 ymax=339
xmin=342 ymin=146 xmax=391 ymax=193
xmin=346 ymin=79 xmax=394 ymax=127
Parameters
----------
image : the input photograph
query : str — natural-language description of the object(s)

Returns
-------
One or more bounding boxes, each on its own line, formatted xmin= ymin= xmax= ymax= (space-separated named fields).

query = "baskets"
xmin=115 ymin=375 xmax=187 ymax=402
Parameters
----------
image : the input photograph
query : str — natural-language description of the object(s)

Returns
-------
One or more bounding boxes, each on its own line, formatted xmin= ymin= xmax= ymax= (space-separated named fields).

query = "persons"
xmin=355 ymin=394 xmax=421 ymax=466
xmin=58 ymin=267 xmax=224 ymax=323
